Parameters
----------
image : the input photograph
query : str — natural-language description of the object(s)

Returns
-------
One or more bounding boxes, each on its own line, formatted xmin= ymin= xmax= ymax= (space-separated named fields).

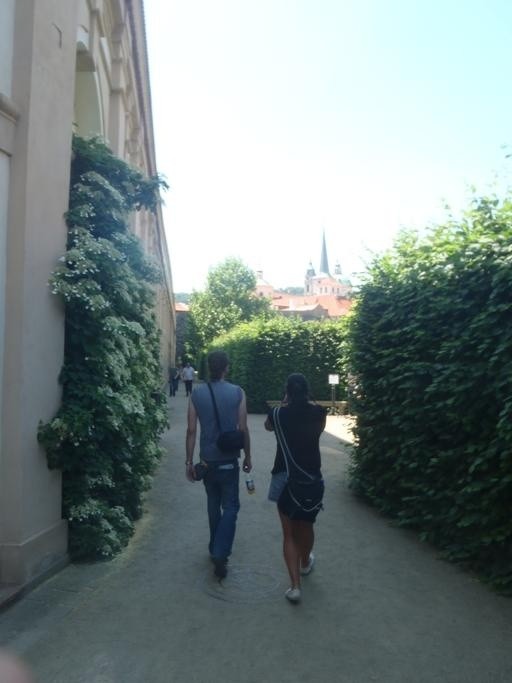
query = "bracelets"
xmin=185 ymin=462 xmax=193 ymax=465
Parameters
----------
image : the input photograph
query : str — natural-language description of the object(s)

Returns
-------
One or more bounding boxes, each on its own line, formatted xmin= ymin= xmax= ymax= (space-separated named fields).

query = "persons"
xmin=167 ymin=362 xmax=195 ymax=397
xmin=265 ymin=373 xmax=326 ymax=601
xmin=186 ymin=351 xmax=252 ymax=577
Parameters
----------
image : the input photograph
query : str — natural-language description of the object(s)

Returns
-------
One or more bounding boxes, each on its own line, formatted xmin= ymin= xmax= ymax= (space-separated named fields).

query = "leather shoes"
xmin=300 ymin=552 xmax=314 ymax=574
xmin=285 ymin=587 xmax=301 ymax=600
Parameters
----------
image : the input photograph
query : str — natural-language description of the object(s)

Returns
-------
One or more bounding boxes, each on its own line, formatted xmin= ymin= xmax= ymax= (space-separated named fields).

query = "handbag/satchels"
xmin=278 ymin=471 xmax=324 ymax=521
xmin=217 ymin=430 xmax=245 ymax=450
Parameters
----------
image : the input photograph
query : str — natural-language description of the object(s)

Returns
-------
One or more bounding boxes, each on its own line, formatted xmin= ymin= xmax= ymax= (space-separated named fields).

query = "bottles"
xmin=244 ymin=467 xmax=255 ymax=494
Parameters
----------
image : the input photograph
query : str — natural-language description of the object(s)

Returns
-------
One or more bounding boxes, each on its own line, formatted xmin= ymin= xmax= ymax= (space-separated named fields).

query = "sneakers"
xmin=215 ymin=553 xmax=226 ymax=576
